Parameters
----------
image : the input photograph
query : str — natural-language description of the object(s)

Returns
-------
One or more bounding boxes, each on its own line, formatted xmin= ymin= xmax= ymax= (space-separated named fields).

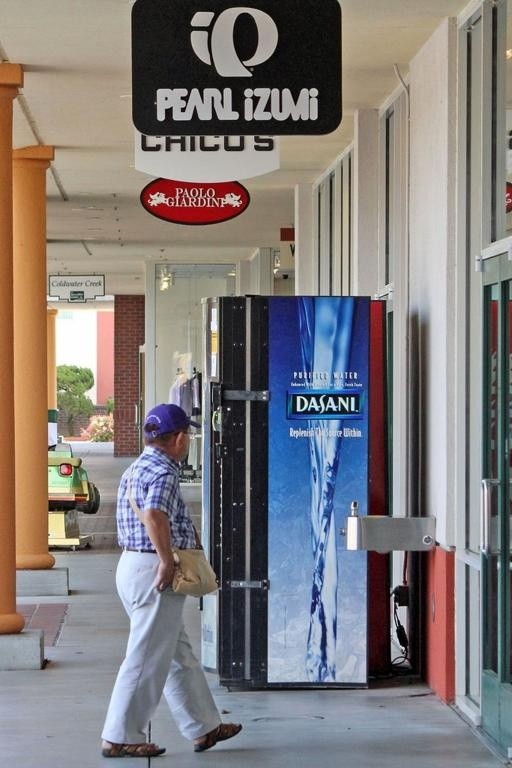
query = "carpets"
xmin=17 ymin=603 xmax=68 ymax=646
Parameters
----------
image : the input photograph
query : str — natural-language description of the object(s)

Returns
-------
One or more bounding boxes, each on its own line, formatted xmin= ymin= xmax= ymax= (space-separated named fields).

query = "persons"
xmin=99 ymin=403 xmax=244 ymax=758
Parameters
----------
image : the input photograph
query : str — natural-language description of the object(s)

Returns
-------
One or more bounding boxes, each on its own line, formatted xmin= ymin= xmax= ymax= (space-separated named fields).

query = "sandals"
xmin=193 ymin=723 xmax=242 ymax=752
xmin=102 ymin=739 xmax=166 ymax=757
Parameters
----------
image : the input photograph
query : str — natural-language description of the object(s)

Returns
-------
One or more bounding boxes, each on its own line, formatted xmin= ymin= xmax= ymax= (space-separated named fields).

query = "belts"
xmin=126 ymin=549 xmax=157 ymax=552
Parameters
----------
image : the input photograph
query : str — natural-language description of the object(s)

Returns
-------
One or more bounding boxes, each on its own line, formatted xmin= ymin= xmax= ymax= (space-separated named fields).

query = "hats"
xmin=144 ymin=404 xmax=201 ymax=437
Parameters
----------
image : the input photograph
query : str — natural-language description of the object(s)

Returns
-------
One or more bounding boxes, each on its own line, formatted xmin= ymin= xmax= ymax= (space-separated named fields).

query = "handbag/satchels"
xmin=171 ymin=545 xmax=217 ymax=596
